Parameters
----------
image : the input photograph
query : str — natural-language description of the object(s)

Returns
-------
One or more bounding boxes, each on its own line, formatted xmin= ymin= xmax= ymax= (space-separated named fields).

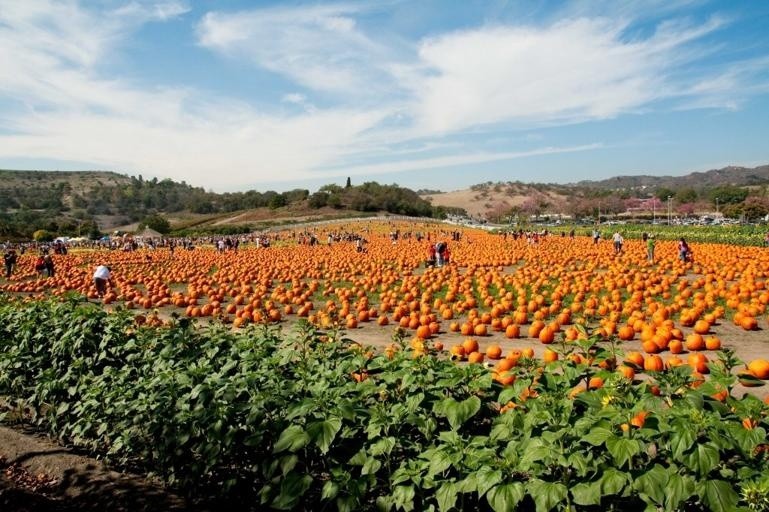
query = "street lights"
xmin=599 ymin=201 xmax=602 ymax=224
xmin=716 ymin=197 xmax=719 ymax=218
xmin=668 ymin=196 xmax=674 ymax=226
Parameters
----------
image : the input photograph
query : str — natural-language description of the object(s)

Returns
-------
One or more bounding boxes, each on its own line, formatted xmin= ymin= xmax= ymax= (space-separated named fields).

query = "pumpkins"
xmin=0 ymin=221 xmax=769 ymax=452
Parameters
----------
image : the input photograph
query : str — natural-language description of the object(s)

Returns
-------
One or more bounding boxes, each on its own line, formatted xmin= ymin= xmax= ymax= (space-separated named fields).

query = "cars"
xmin=604 ymin=217 xmax=732 ymax=226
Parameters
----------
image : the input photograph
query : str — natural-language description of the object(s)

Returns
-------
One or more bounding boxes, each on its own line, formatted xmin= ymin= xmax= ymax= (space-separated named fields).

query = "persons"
xmin=618 ymin=232 xmax=625 ymax=249
xmin=646 ymin=230 xmax=653 ymax=240
xmin=593 ymin=228 xmax=600 ymax=244
xmin=91 ymin=263 xmax=114 ymax=298
xmin=434 ymin=241 xmax=443 ymax=268
xmin=0 ymin=233 xmax=69 ymax=281
xmin=611 ymin=230 xmax=622 ymax=253
xmin=646 ymin=234 xmax=657 ymax=262
xmin=610 ymin=232 xmax=615 ymax=248
xmin=642 ymin=230 xmax=647 ymax=241
xmin=494 ymin=225 xmax=576 ymax=244
xmin=441 ymin=241 xmax=450 ymax=265
xmin=425 ymin=243 xmax=436 ymax=269
xmin=678 ymin=237 xmax=688 ymax=262
